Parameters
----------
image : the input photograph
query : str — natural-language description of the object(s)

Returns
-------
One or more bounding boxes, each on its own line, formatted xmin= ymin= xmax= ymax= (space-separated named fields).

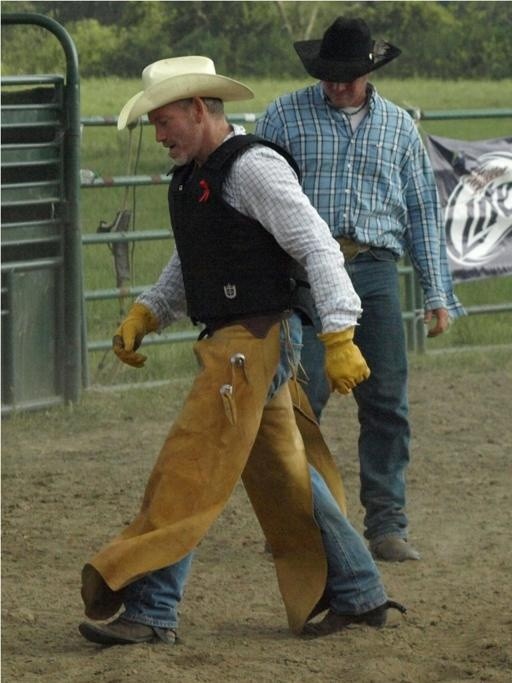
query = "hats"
xmin=294 ymin=17 xmax=401 ymax=82
xmin=117 ymin=55 xmax=254 ymax=130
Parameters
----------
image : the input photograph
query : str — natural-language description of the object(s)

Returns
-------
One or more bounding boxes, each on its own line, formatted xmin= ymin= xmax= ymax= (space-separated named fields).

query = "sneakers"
xmin=80 ymin=619 xmax=174 ymax=644
xmin=370 ymin=538 xmax=420 ymax=561
xmin=303 ymin=606 xmax=385 ymax=634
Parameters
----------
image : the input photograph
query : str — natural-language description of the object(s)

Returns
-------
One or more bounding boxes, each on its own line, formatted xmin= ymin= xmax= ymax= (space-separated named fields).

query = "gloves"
xmin=318 ymin=325 xmax=370 ymax=393
xmin=113 ymin=304 xmax=156 ymax=367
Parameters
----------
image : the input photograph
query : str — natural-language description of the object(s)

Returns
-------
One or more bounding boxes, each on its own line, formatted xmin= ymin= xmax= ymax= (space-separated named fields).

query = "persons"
xmin=255 ymin=14 xmax=463 ymax=562
xmin=77 ymin=53 xmax=400 ymax=640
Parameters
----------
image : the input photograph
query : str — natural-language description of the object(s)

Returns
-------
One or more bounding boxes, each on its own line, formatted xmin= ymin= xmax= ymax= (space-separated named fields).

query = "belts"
xmin=337 ymin=239 xmax=397 ymax=262
